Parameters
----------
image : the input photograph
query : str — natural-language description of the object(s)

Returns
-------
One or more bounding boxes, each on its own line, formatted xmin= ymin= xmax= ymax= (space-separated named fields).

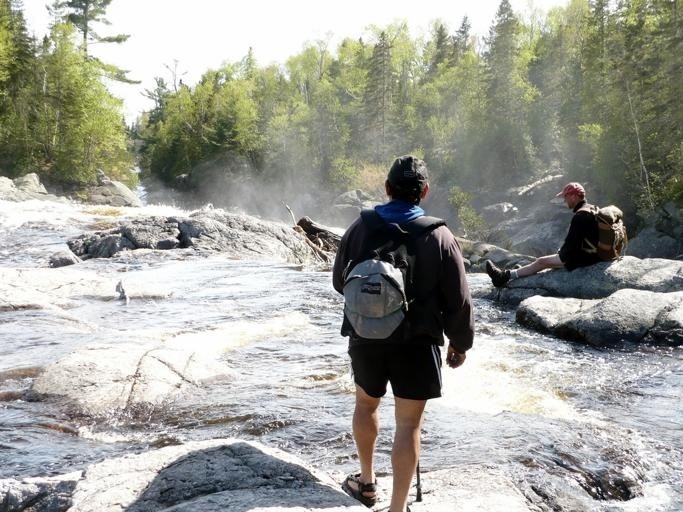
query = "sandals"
xmin=342 ymin=473 xmax=378 ymax=506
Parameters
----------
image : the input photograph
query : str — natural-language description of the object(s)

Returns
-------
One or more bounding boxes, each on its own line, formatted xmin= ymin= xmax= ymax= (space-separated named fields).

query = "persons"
xmin=333 ymin=156 xmax=474 ymax=512
xmin=486 ymin=183 xmax=600 ymax=289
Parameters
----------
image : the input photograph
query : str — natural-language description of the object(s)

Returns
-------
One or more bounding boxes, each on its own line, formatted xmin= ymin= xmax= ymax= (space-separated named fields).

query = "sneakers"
xmin=485 ymin=259 xmax=510 ymax=286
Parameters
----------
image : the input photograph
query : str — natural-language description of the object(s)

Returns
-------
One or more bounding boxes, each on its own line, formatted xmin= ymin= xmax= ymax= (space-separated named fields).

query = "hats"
xmin=388 ymin=155 xmax=428 ymax=194
xmin=556 ymin=183 xmax=585 ymax=196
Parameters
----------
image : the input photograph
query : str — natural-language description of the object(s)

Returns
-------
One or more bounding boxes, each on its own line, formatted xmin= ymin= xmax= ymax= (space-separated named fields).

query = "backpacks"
xmin=579 ymin=205 xmax=628 ymax=262
xmin=339 ymin=211 xmax=446 ymax=341
